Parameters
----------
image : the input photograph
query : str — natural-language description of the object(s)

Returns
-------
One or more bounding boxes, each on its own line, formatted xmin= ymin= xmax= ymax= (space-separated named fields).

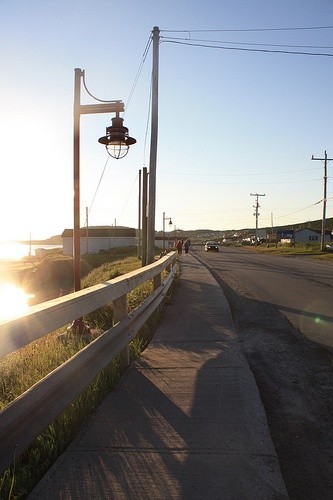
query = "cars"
xmin=204 ymin=240 xmax=219 ymax=253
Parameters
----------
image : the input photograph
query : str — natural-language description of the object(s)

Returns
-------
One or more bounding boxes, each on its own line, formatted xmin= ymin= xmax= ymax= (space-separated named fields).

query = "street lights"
xmin=66 ymin=67 xmax=137 ymax=334
xmin=162 ymin=212 xmax=173 ymax=254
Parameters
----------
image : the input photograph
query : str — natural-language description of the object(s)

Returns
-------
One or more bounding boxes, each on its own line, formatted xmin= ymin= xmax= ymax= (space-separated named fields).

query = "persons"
xmin=183 ymin=239 xmax=191 ymax=256
xmin=176 ymin=240 xmax=183 ymax=256
xmin=65 ymin=316 xmax=93 ymax=354
